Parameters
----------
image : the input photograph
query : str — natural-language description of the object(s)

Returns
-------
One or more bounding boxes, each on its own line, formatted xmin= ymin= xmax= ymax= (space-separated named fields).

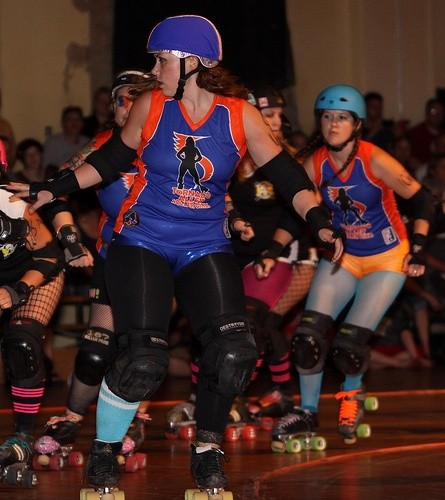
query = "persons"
xmin=163 ymin=174 xmax=258 ymax=431
xmin=233 ymin=85 xmax=320 ymax=420
xmin=0 ymin=86 xmax=445 ymax=380
xmin=33 ymin=71 xmax=164 ymax=455
xmin=0 ymin=138 xmax=66 ymax=469
xmin=253 ymin=84 xmax=432 ymax=441
xmin=0 ymin=14 xmax=344 ymax=490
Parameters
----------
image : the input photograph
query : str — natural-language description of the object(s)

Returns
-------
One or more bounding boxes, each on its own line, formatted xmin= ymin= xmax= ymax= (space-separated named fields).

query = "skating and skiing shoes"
xmin=0 ymin=431 xmax=37 ymax=487
xmin=248 ymin=391 xmax=294 ymax=432
xmin=223 ymin=396 xmax=257 ymax=441
xmin=162 ymin=402 xmax=196 ymax=438
xmin=31 ymin=408 xmax=84 ymax=468
xmin=336 ymin=382 xmax=380 ymax=443
xmin=272 ymin=407 xmax=326 ymax=452
xmin=184 ymin=445 xmax=233 ymax=500
xmin=79 ymin=438 xmax=126 ymax=500
xmin=117 ymin=412 xmax=151 ymax=471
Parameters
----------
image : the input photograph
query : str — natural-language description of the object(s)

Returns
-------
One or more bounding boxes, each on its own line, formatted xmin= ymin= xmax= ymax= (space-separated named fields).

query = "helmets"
xmin=112 ymin=67 xmax=155 ymax=95
xmin=250 ymin=85 xmax=285 ymax=109
xmin=146 ymin=13 xmax=223 ymax=61
xmin=315 ymin=84 xmax=367 ymax=128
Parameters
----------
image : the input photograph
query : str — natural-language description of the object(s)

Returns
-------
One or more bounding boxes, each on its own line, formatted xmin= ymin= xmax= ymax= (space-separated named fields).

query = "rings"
xmin=413 ymin=269 xmax=418 ymax=273
